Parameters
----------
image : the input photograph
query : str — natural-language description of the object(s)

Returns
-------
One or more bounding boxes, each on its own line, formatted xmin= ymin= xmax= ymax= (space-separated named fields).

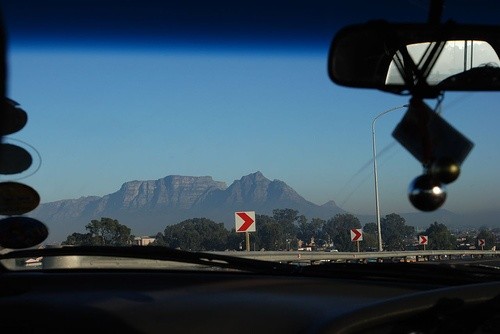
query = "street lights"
xmin=373 ymin=104 xmax=410 ymax=253
